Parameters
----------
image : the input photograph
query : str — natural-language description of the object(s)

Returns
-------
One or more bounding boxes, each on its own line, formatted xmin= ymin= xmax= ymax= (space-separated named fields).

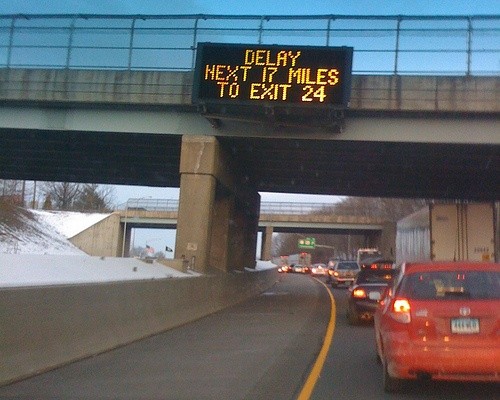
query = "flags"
xmin=145 ymin=245 xmax=154 ymax=254
xmin=165 ymin=246 xmax=172 ymax=253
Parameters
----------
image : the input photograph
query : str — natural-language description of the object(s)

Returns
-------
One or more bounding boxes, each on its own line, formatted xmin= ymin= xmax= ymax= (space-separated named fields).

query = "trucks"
xmin=272 ymin=252 xmax=311 ymax=274
xmin=390 ymin=201 xmax=497 ymax=274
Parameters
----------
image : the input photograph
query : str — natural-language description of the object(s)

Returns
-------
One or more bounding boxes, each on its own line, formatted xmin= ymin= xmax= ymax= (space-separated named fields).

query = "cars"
xmin=344 ymin=260 xmax=400 ymax=325
xmin=311 ymin=263 xmax=327 ymax=277
xmin=368 ymin=259 xmax=500 ymax=393
xmin=325 ymin=257 xmax=362 ymax=288
